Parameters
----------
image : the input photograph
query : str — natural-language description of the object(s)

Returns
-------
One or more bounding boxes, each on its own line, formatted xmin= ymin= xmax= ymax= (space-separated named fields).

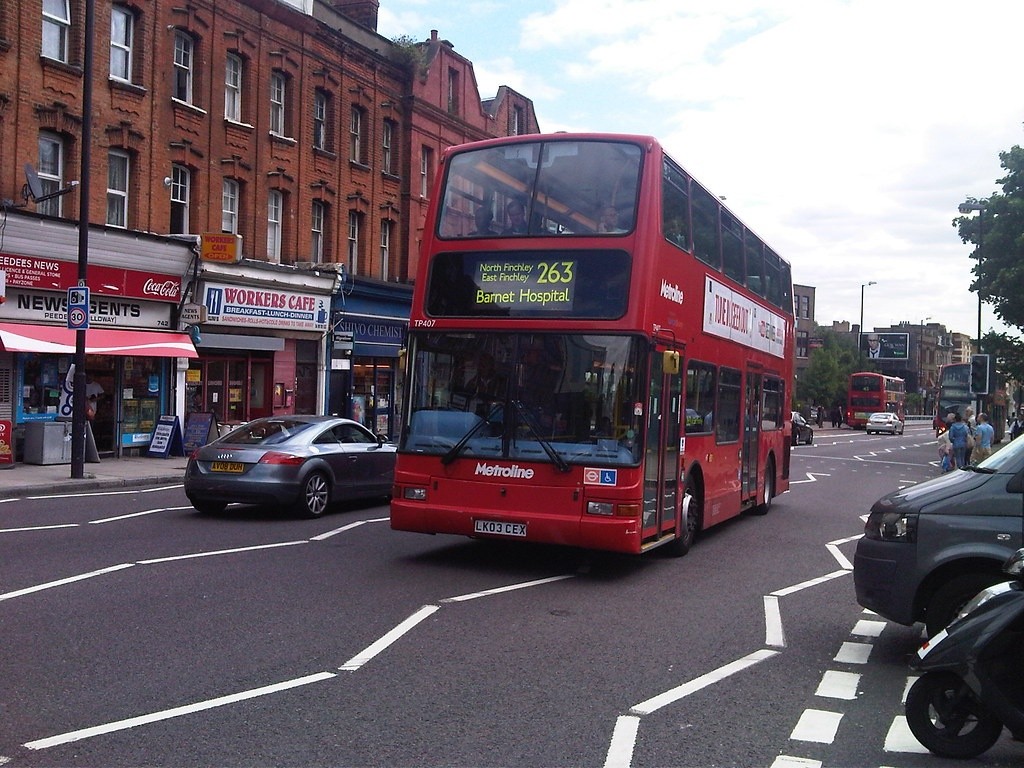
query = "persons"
xmin=815 ymin=404 xmax=844 ymax=429
xmin=1006 ymin=410 xmax=1024 ymax=441
xmin=664 ymin=217 xmax=687 ymax=249
xmin=598 ymin=206 xmax=628 ymax=235
xmin=934 ymin=406 xmax=998 ymax=472
xmin=467 ymin=207 xmax=498 ymax=235
xmin=85 ymin=371 xmax=105 ymax=430
xmin=500 ymin=201 xmax=529 ymax=236
xmin=463 ymin=353 xmax=507 ymax=400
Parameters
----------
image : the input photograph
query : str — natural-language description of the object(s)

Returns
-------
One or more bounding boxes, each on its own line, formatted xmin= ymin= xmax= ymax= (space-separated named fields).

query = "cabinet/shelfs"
xmin=23 ymin=422 xmax=87 ymax=465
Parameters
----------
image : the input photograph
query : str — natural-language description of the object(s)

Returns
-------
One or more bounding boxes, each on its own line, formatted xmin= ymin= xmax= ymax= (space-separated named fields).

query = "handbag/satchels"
xmin=966 ymin=435 xmax=975 ymax=449
xmin=86 ymin=401 xmax=95 ymax=420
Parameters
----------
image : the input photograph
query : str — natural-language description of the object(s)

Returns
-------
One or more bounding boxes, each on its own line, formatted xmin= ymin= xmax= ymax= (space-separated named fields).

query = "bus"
xmin=845 ymin=372 xmax=907 ymax=430
xmin=390 ymin=130 xmax=798 ymax=559
xmin=932 ymin=361 xmax=1007 ymax=446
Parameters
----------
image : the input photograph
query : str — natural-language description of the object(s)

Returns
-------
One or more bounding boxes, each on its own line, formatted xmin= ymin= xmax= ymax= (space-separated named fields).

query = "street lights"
xmin=859 ymin=281 xmax=877 ymax=372
xmin=918 ymin=317 xmax=931 ymax=420
xmin=958 ymin=202 xmax=985 ymax=423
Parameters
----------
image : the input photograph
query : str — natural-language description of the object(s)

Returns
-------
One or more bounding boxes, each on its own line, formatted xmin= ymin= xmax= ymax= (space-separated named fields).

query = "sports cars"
xmin=184 ymin=414 xmax=398 ymax=520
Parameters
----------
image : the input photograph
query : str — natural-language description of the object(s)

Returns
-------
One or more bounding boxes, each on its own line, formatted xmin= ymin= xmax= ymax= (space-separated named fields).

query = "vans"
xmin=853 ymin=431 xmax=1024 ymax=641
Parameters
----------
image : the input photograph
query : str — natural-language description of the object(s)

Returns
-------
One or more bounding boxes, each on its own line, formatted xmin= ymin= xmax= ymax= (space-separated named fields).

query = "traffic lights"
xmin=970 ymin=353 xmax=990 ymax=394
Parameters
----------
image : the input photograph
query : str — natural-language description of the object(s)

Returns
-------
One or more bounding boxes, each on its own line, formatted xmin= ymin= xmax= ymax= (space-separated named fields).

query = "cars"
xmin=866 ymin=412 xmax=904 ymax=435
xmin=790 ymin=411 xmax=814 ymax=447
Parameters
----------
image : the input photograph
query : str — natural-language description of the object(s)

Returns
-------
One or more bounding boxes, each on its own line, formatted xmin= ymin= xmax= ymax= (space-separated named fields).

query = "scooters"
xmin=904 ymin=549 xmax=1024 ymax=761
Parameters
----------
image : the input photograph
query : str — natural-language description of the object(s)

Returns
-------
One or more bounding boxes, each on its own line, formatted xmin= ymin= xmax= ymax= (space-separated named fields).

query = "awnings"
xmin=0 ymin=319 xmax=199 ymax=358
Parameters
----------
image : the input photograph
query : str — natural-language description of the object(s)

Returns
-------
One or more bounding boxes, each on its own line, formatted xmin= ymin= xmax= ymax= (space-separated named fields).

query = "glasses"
xmin=606 ymin=213 xmax=620 ymax=218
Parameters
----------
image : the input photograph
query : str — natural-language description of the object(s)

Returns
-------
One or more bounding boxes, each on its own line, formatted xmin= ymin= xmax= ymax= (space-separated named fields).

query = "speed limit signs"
xmin=69 ymin=307 xmax=86 ymax=327
